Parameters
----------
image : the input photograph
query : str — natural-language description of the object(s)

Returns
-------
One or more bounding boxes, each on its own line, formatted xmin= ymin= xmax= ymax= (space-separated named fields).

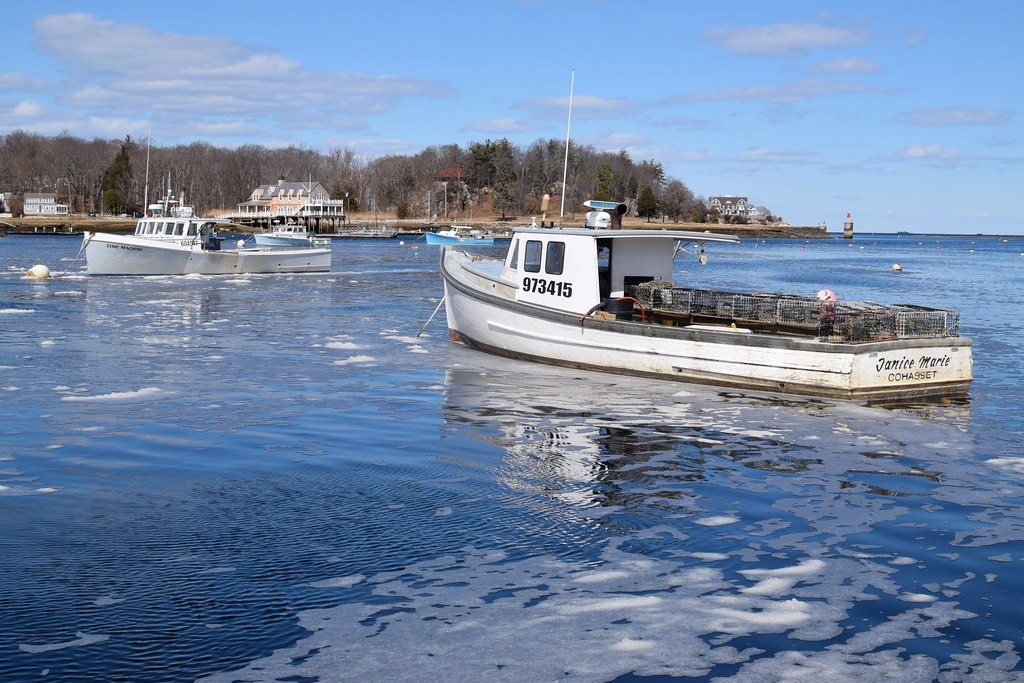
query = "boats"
xmin=84 ymin=129 xmax=333 ymax=278
xmin=416 ymin=70 xmax=976 ymax=404
xmin=424 ymin=224 xmax=495 ymax=246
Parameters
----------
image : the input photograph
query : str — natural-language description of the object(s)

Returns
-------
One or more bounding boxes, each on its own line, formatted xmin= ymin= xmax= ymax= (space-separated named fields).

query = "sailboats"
xmin=335 ymin=192 xmax=406 ymax=239
